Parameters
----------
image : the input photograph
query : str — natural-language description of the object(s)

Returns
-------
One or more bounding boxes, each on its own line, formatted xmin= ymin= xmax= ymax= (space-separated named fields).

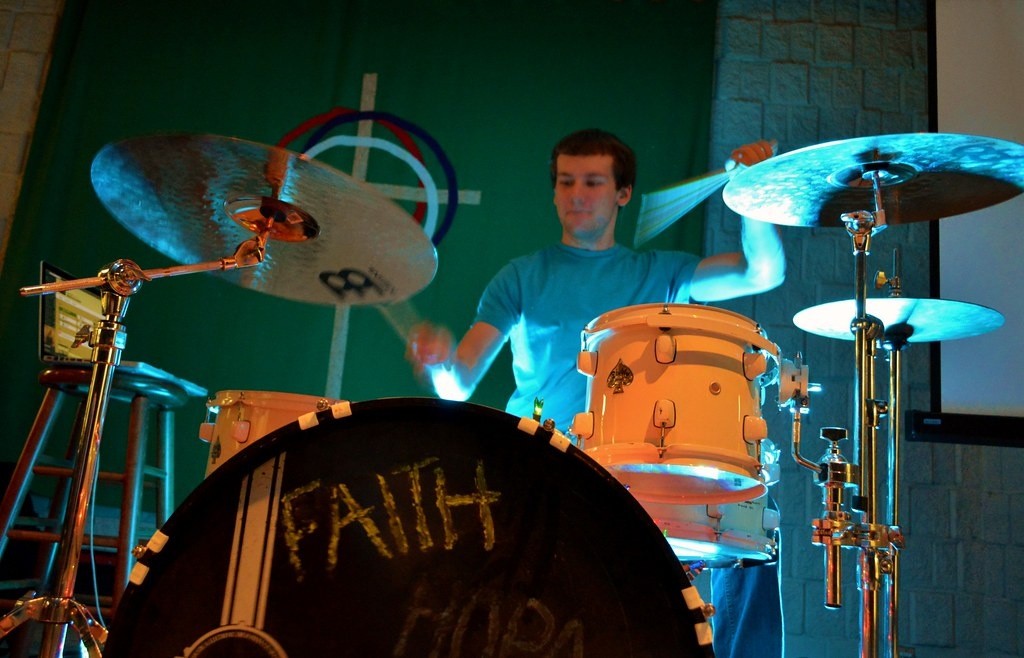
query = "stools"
xmin=0 ymin=361 xmax=209 ymax=626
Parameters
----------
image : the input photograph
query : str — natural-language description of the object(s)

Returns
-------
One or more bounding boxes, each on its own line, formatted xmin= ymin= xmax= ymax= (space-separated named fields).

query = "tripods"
xmin=0 ymin=214 xmax=282 ymax=658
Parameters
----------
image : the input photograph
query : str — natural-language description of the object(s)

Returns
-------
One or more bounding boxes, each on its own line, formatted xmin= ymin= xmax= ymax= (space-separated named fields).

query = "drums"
xmin=570 ymin=303 xmax=783 ymax=506
xmin=638 ymin=439 xmax=781 ymax=570
xmin=198 ymin=390 xmax=349 ymax=479
xmin=102 ymin=396 xmax=716 ymax=658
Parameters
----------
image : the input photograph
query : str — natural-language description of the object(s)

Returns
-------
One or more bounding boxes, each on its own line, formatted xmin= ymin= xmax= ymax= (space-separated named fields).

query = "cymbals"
xmin=721 ymin=133 xmax=1024 ymax=229
xmin=792 ymin=298 xmax=1006 ymax=344
xmin=89 ymin=132 xmax=441 ymax=306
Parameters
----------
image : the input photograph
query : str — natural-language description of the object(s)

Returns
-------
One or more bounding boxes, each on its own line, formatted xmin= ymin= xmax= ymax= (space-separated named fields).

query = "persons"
xmin=405 ymin=128 xmax=784 ymax=658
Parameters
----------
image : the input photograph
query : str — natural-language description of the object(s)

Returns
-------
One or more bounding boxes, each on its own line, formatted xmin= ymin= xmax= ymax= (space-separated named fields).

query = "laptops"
xmin=38 ymin=260 xmax=208 ymax=399
